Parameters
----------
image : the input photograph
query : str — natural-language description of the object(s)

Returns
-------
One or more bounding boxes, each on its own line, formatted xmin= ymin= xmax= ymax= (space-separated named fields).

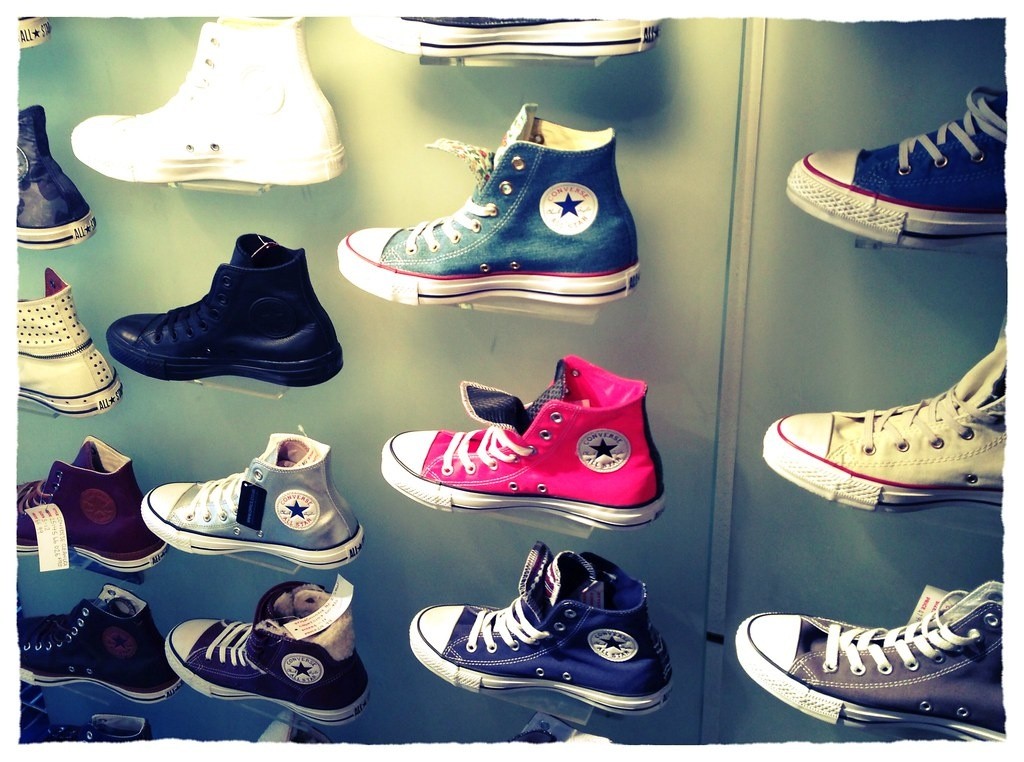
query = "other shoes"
xmin=18 ymin=17 xmax=51 ymax=48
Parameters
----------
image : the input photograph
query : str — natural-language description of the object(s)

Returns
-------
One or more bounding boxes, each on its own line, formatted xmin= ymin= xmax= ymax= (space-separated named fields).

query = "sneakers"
xmin=350 ymin=17 xmax=662 ymax=57
xmin=785 ymin=86 xmax=1007 ymax=252
xmin=409 ymin=541 xmax=672 ymax=716
xmin=20 ymin=714 xmax=152 ymax=742
xmin=336 ymin=103 xmax=641 ymax=305
xmin=18 ymin=267 xmax=123 ymax=418
xmin=71 ymin=17 xmax=347 ymax=185
xmin=17 ymin=583 xmax=184 ymax=704
xmin=164 ymin=581 xmax=370 ymax=726
xmin=735 ymin=579 xmax=1006 ymax=741
xmin=16 ymin=435 xmax=169 ymax=572
xmin=763 ymin=314 xmax=1006 ymax=513
xmin=16 ymin=105 xmax=97 ymax=250
xmin=381 ymin=353 xmax=665 ymax=531
xmin=140 ymin=432 xmax=364 ymax=570
xmin=106 ymin=234 xmax=343 ymax=387
